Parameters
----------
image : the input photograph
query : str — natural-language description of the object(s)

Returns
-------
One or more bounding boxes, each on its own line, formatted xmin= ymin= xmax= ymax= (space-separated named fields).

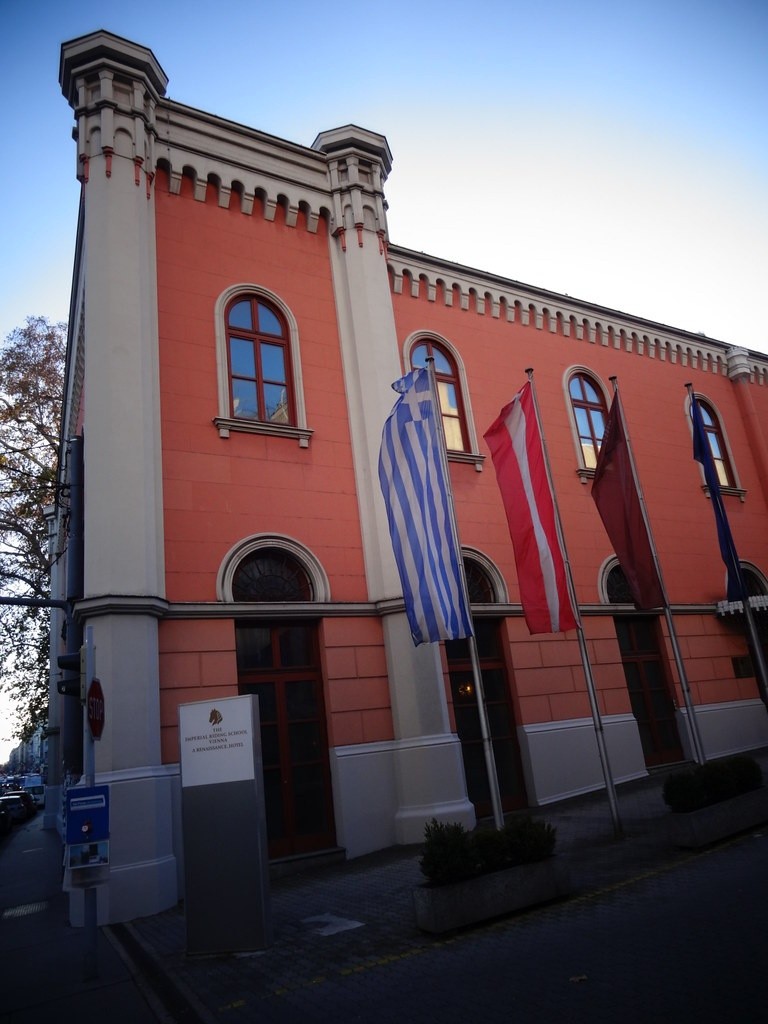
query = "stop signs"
xmin=87 ymin=677 xmax=106 ymax=741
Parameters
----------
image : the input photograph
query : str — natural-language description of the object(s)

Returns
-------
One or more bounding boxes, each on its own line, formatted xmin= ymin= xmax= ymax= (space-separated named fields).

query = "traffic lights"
xmin=55 ymin=650 xmax=81 ymax=700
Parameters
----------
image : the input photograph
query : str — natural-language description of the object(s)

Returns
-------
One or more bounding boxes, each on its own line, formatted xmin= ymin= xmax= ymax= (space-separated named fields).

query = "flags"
xmin=688 ymin=396 xmax=750 ymax=609
xmin=482 ymin=380 xmax=578 ymax=635
xmin=378 ymin=366 xmax=473 ymax=646
xmin=591 ymin=389 xmax=667 ymax=610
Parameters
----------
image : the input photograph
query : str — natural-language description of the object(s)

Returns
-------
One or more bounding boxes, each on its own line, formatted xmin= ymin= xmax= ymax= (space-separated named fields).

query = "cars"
xmin=0 ymin=773 xmax=44 ymax=835
